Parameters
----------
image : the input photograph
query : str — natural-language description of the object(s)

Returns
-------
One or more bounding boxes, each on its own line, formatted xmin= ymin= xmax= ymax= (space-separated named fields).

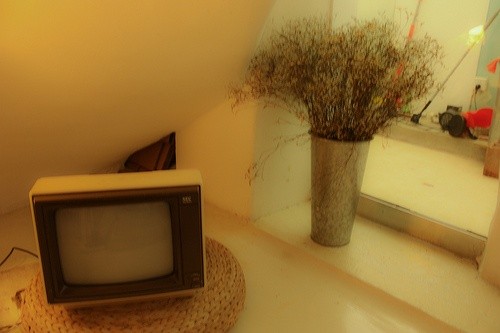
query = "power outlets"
xmin=476 ymin=77 xmax=488 ymax=91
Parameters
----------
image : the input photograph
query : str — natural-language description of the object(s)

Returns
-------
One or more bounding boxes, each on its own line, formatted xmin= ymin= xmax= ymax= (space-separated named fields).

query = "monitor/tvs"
xmin=29 ymin=168 xmax=207 ymax=309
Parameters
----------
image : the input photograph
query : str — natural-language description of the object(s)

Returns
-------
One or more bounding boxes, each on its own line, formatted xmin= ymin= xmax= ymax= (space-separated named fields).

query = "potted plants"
xmin=227 ymin=7 xmax=447 ymax=248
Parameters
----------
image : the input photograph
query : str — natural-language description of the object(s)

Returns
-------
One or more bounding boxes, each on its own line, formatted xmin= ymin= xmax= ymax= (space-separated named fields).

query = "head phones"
xmin=437 ymin=110 xmax=479 ymax=141
